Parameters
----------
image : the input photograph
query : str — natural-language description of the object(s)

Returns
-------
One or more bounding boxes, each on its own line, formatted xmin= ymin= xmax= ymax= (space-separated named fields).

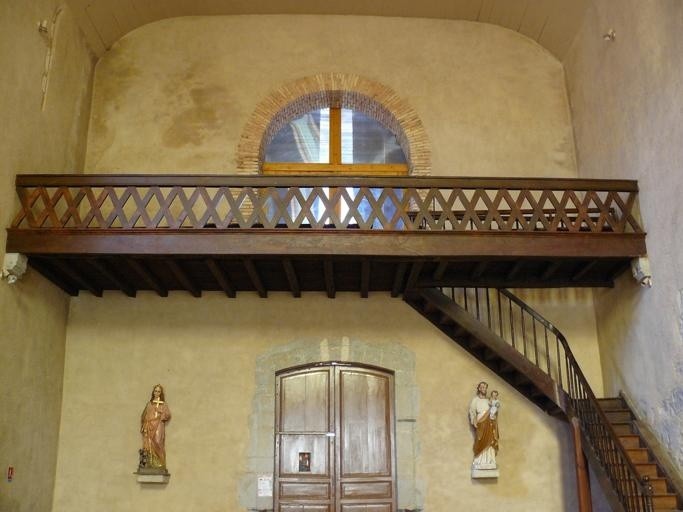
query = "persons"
xmin=469 ymin=382 xmax=499 ymax=466
xmin=139 ymin=383 xmax=171 ymax=473
xmin=487 ymin=390 xmax=499 ymax=407
